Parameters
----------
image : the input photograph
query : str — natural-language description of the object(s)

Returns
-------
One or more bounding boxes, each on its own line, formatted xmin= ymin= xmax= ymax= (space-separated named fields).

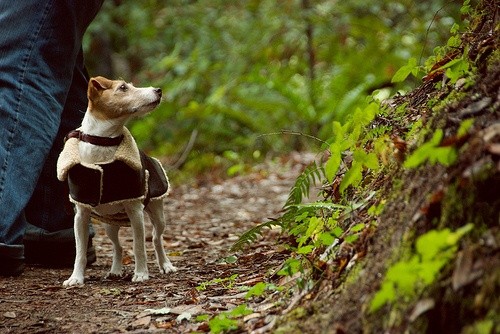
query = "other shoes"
xmin=0 ymin=244 xmax=23 ymax=276
xmin=23 ymin=240 xmax=96 ymax=268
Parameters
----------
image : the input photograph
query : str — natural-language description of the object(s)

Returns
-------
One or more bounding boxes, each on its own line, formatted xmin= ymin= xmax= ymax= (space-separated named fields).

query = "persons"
xmin=1 ymin=0 xmax=100 ymax=277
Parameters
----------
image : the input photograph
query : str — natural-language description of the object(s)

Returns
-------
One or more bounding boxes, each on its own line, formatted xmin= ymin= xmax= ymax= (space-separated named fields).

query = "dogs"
xmin=61 ymin=75 xmax=178 ymax=290
